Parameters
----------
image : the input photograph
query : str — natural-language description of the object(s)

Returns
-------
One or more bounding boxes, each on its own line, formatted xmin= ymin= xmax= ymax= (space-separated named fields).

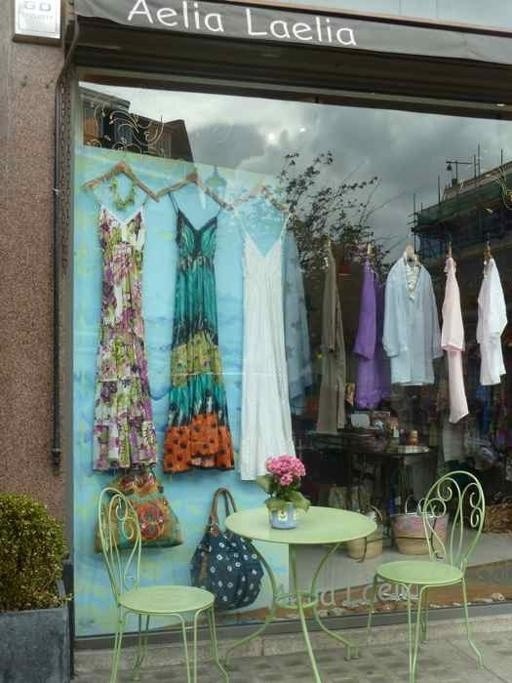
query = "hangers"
xmin=78 ymin=144 xmax=294 ymax=214
xmin=322 ymin=230 xmax=499 ymax=267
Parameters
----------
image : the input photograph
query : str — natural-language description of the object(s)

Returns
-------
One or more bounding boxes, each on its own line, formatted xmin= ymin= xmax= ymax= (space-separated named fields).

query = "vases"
xmin=268 ymin=501 xmax=299 ymax=529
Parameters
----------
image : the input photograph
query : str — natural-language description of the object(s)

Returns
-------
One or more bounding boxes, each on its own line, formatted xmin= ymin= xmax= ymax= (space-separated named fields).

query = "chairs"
xmin=96 ymin=483 xmax=228 ymax=683
xmin=364 ymin=468 xmax=488 ymax=683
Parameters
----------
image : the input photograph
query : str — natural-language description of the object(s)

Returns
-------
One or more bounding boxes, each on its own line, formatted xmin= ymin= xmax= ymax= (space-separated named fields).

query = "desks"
xmin=309 ymin=433 xmax=443 ymax=563
xmin=223 ymin=504 xmax=378 ymax=682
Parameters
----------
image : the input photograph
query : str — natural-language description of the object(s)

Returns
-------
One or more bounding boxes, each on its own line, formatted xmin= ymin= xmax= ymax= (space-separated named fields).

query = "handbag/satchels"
xmin=191 ymin=487 xmax=263 ymax=611
xmin=95 ymin=464 xmax=182 ymax=552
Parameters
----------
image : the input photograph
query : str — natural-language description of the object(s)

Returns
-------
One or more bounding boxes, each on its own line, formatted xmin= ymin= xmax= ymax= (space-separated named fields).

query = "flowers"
xmin=255 ymin=453 xmax=312 ymax=515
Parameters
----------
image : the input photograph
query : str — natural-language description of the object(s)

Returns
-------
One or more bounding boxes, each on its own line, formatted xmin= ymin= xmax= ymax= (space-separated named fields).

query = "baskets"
xmin=345 ymin=504 xmax=383 ymax=559
xmin=390 ymin=494 xmax=450 ymax=555
xmin=484 ymin=504 xmax=512 ymax=536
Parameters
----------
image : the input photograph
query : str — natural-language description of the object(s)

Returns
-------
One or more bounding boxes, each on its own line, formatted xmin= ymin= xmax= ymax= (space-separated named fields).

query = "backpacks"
xmin=338 ymin=428 xmax=390 ymax=452
